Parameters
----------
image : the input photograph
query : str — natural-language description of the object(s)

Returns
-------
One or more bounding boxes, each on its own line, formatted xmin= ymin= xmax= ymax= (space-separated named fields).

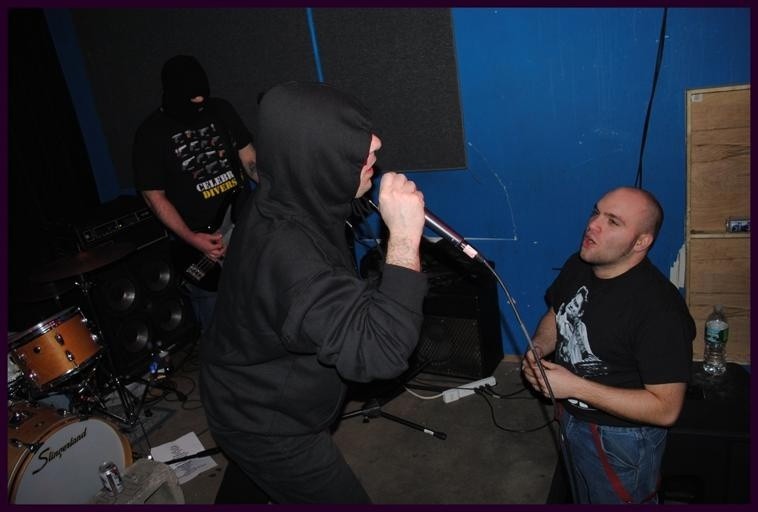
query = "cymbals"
xmin=43 ymin=242 xmax=138 ymax=281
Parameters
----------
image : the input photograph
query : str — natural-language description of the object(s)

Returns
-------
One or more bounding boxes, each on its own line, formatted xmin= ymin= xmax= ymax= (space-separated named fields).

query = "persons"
xmin=131 ymin=55 xmax=259 ymax=330
xmin=523 ymin=187 xmax=694 ymax=502
xmin=199 ymin=85 xmax=425 ymax=503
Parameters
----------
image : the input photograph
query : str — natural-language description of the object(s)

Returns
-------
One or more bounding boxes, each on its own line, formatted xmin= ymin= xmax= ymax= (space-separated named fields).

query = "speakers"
xmin=70 ymin=192 xmax=202 ymax=377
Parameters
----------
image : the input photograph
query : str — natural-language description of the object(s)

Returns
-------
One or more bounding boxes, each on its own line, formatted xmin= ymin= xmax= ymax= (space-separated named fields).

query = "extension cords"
xmin=443 ymin=376 xmax=496 ymax=403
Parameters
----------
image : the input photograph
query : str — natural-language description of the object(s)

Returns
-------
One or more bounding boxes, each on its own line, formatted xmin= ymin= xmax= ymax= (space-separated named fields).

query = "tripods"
xmin=78 ymin=273 xmax=188 ymax=448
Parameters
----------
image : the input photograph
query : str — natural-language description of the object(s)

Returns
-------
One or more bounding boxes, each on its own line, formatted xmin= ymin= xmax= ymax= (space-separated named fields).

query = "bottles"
xmin=703 ymin=305 xmax=728 ymax=375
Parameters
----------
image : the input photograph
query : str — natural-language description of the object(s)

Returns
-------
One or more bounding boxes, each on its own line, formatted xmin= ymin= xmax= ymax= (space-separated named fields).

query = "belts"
xmin=559 ymin=399 xmax=639 ymax=428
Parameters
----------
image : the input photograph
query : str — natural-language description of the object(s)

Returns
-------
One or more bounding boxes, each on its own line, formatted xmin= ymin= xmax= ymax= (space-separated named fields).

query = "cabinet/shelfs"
xmin=685 ymin=84 xmax=751 ymax=365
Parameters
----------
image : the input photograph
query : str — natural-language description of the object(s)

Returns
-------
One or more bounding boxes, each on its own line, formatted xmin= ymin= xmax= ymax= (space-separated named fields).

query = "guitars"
xmin=167 ymin=159 xmax=260 ymax=293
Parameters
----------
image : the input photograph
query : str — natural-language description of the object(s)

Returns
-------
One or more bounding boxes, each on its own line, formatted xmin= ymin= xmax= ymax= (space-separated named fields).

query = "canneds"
xmin=99 ymin=461 xmax=125 ymax=497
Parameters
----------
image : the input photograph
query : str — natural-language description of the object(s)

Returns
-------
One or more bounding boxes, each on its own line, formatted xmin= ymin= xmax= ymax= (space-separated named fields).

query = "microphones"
xmin=378 ymin=169 xmax=478 ymax=259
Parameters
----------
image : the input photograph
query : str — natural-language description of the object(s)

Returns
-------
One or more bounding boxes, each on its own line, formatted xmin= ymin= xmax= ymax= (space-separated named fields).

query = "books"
xmin=150 ymin=432 xmax=218 ymax=485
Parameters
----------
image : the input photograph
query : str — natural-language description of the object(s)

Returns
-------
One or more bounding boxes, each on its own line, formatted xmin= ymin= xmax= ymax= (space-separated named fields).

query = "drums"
xmin=6 ymin=400 xmax=132 ymax=503
xmin=8 ymin=305 xmax=103 ymax=390
